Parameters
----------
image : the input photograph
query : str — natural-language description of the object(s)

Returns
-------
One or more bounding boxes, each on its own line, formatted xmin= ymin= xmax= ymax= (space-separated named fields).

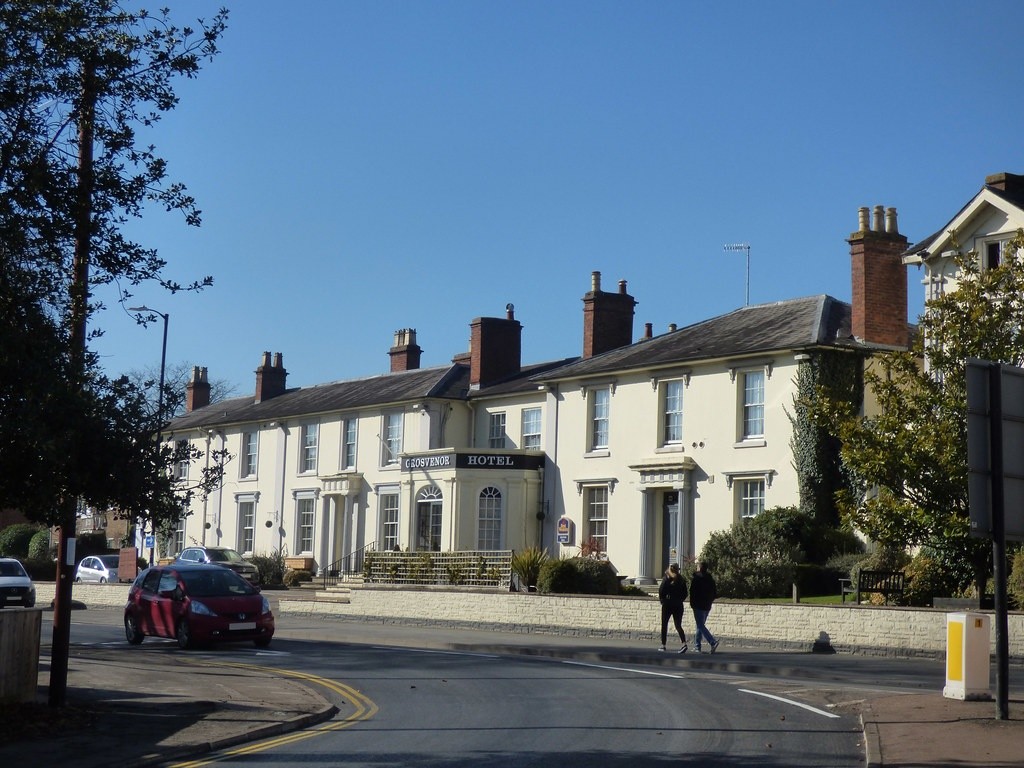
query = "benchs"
xmin=838 ymin=569 xmax=905 ymax=606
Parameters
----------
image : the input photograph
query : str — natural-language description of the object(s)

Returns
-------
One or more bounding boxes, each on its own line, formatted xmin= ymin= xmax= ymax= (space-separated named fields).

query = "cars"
xmin=76 ymin=555 xmax=143 ymax=584
xmin=173 ymin=547 xmax=261 ymax=593
xmin=123 ymin=560 xmax=276 ymax=649
xmin=0 ymin=556 xmax=36 ymax=608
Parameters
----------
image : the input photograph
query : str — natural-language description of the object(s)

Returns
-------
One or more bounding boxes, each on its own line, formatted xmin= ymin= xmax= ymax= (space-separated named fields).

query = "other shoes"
xmin=691 ymin=647 xmax=700 ymax=652
xmin=708 ymin=641 xmax=719 ymax=654
xmin=678 ymin=645 xmax=687 ymax=654
xmin=657 ymin=647 xmax=666 ymax=652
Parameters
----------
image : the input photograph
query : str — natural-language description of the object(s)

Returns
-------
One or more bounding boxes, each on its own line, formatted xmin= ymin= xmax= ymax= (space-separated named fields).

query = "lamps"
xmin=265 ymin=510 xmax=278 ymax=528
xmin=205 ymin=513 xmax=217 ymax=530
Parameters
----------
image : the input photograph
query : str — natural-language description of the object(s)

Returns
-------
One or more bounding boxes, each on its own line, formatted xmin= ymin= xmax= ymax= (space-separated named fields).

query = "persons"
xmin=689 ymin=560 xmax=720 ymax=655
xmin=657 ymin=562 xmax=688 ymax=654
xmin=390 ymin=544 xmax=402 ymax=551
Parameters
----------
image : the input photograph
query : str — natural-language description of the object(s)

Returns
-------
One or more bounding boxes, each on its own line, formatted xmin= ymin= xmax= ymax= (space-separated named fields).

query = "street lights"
xmin=127 ymin=307 xmax=169 ymax=568
